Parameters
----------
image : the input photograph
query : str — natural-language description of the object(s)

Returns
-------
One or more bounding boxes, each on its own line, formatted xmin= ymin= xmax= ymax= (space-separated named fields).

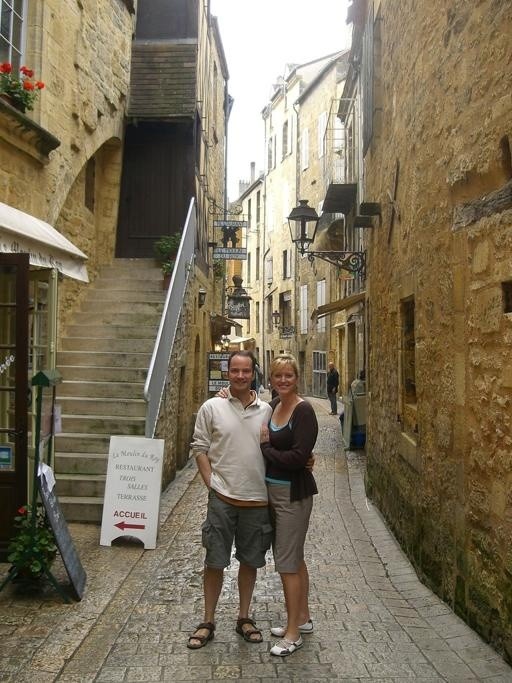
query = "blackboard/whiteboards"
xmin=38 ymin=472 xmax=87 ymax=601
xmin=100 ymin=435 xmax=165 ymax=550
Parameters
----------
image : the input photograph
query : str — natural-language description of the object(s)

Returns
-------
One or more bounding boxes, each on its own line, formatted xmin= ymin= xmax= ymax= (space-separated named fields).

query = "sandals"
xmin=269 ymin=618 xmax=314 ymax=637
xmin=235 ymin=615 xmax=264 ymax=643
xmin=268 ymin=634 xmax=304 ymax=656
xmin=186 ymin=621 xmax=216 ymax=649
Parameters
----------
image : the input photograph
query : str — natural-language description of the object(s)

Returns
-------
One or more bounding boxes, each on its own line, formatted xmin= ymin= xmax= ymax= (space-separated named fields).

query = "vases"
xmin=0 ymin=91 xmax=14 ymax=104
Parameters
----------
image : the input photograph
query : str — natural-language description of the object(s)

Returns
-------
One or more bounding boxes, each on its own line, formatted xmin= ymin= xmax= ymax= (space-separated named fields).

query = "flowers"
xmin=0 ymin=60 xmax=45 ymax=106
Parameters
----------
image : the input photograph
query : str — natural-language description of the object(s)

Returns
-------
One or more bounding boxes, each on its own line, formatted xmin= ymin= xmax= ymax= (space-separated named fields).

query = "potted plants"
xmin=6 ymin=502 xmax=57 ymax=585
xmin=155 ymin=231 xmax=183 ymax=288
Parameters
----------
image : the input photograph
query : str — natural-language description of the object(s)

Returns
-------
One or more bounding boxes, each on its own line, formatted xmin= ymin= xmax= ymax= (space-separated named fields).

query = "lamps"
xmin=284 ymin=199 xmax=367 ymax=282
xmin=272 ymin=309 xmax=294 ymax=334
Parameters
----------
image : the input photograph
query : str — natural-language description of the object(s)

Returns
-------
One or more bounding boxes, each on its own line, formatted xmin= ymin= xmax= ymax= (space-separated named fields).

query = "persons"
xmin=327 ymin=361 xmax=339 ymax=415
xmin=213 ymin=353 xmax=319 ymax=656
xmin=187 ymin=351 xmax=315 ymax=649
